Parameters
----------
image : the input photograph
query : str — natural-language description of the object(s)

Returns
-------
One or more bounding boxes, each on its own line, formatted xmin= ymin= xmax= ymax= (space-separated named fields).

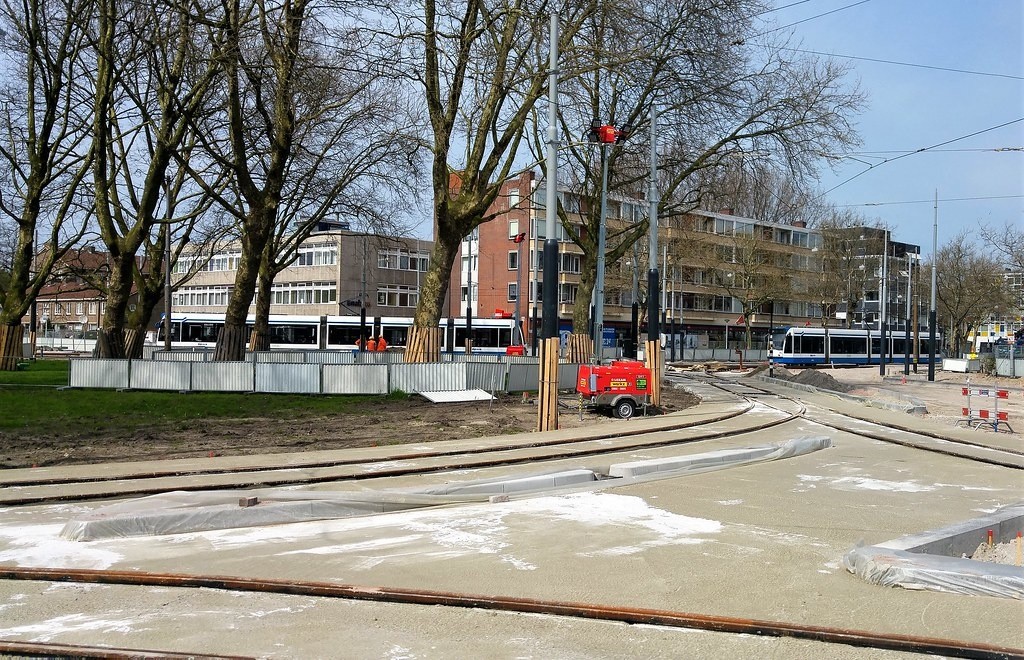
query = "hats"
xmin=378 ymin=335 xmax=383 ymax=338
xmin=369 ymin=336 xmax=374 ymax=340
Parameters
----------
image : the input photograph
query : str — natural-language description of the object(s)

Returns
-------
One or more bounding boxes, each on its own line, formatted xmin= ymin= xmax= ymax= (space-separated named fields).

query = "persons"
xmin=355 ymin=335 xmax=386 ymax=353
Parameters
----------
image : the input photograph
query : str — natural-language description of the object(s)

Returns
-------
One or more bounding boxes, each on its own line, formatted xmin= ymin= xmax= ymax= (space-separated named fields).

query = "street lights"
xmin=513 ymin=232 xmax=527 ymax=346
xmin=584 ymin=117 xmax=632 ymax=367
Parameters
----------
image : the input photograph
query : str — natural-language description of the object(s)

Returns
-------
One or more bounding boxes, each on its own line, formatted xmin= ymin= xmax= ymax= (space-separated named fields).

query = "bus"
xmin=766 ymin=323 xmax=943 ymax=366
xmin=156 ymin=311 xmax=528 ymax=358
